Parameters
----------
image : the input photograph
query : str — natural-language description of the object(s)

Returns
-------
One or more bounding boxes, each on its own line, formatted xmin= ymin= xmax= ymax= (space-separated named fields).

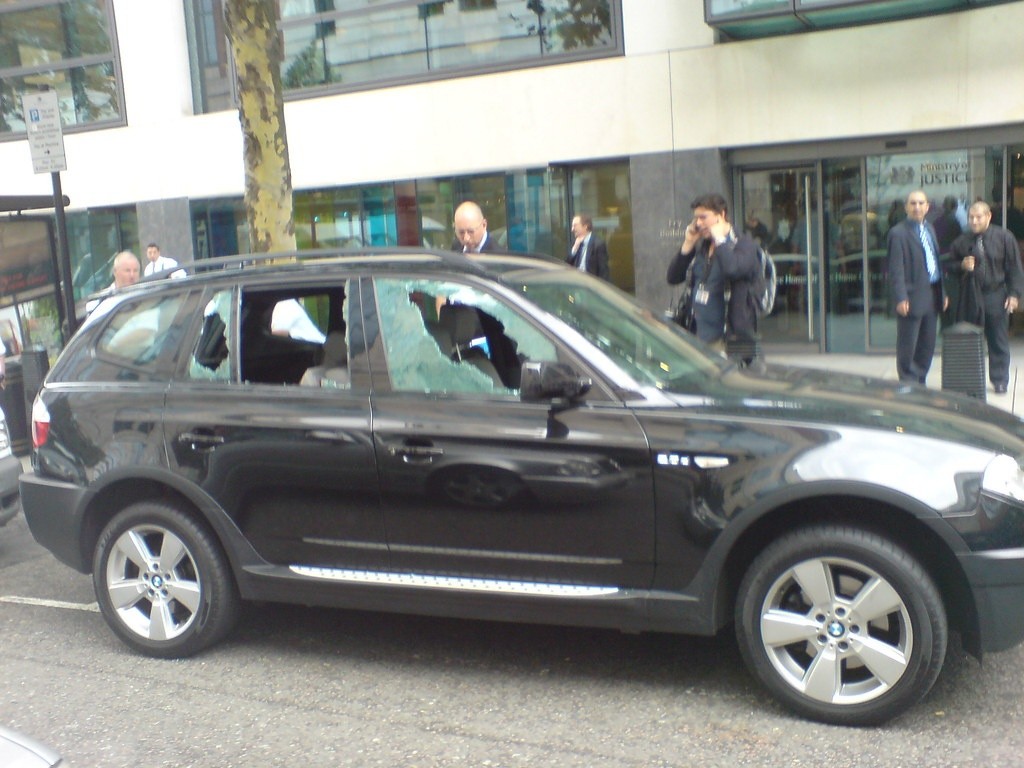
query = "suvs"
xmin=19 ymin=245 xmax=1024 ymax=729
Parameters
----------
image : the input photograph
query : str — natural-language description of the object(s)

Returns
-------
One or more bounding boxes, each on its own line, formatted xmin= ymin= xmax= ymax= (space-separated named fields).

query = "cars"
xmin=0 ymin=408 xmax=24 ymax=528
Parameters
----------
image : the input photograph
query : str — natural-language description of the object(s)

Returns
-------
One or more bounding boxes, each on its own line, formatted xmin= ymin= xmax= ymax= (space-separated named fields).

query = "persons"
xmin=948 ymin=201 xmax=1024 ymax=392
xmin=450 ymin=201 xmax=508 ymax=256
xmin=209 ymin=290 xmax=326 ymax=347
xmin=743 ymin=195 xmax=1024 ymax=337
xmin=562 ymin=214 xmax=609 ymax=283
xmin=90 ymin=251 xmax=158 ymax=355
xmin=884 ymin=189 xmax=950 ymax=385
xmin=144 ymin=244 xmax=187 ymax=281
xmin=666 ymin=192 xmax=764 ymax=365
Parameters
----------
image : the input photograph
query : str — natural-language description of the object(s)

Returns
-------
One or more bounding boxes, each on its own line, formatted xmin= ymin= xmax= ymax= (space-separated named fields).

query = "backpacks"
xmin=742 ymin=238 xmax=778 ymax=317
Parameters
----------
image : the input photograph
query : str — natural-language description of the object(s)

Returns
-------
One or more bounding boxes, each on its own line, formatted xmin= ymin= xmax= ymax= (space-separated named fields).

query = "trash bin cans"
xmin=0 ymin=356 xmax=31 ymax=458
xmin=941 ymin=323 xmax=987 ymax=402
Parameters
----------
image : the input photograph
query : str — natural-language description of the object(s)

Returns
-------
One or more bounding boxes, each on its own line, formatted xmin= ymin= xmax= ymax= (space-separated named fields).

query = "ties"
xmin=977 ymin=236 xmax=987 ymax=286
xmin=708 ymin=240 xmax=714 ymax=258
xmin=576 ymin=242 xmax=583 ymax=266
xmin=919 ymin=225 xmax=936 ymax=276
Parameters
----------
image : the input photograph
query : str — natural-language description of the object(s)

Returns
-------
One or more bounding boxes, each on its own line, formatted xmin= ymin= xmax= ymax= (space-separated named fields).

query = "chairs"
xmin=299 ymin=329 xmax=349 ymax=388
xmin=423 ymin=303 xmax=508 ymax=390
